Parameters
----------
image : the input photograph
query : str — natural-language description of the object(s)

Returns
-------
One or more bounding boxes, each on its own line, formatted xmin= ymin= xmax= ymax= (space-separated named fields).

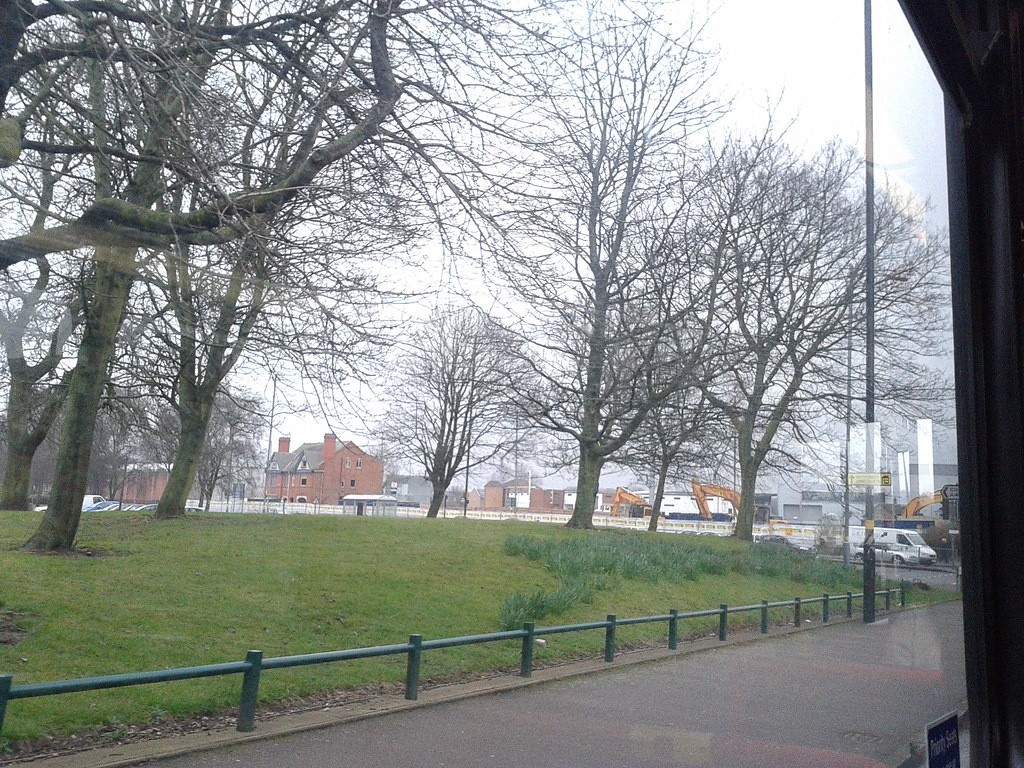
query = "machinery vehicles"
xmin=692 ymin=478 xmax=771 ymax=525
xmin=610 ymin=486 xmax=654 ymax=519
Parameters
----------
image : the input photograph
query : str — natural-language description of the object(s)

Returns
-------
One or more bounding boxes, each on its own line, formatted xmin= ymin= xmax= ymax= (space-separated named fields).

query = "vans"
xmin=849 ymin=526 xmax=938 ymax=567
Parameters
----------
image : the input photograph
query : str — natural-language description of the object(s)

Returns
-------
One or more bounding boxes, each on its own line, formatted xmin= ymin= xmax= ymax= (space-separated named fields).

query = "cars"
xmin=855 ymin=541 xmax=919 ymax=566
